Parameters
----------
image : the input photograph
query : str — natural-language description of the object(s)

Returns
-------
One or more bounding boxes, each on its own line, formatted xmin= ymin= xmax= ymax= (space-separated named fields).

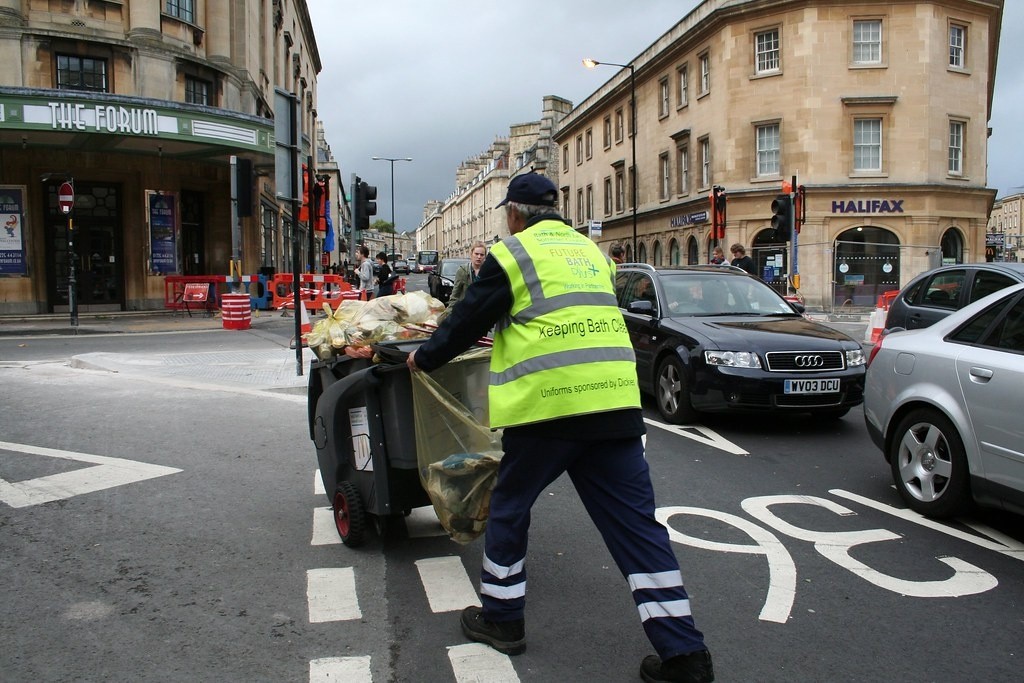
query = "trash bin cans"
xmin=308 ymin=337 xmax=531 ymax=553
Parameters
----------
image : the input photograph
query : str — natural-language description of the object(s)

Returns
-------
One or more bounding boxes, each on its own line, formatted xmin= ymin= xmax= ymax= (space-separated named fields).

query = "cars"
xmin=864 ymin=283 xmax=1024 ymax=515
xmin=886 ymin=261 xmax=1024 ymax=330
xmin=428 ymin=258 xmax=471 ymax=300
xmin=392 ymin=259 xmax=409 ymax=275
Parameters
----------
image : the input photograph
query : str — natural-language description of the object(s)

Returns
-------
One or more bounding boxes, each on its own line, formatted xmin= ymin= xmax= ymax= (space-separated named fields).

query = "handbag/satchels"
xmin=378 ymin=265 xmax=398 ymax=283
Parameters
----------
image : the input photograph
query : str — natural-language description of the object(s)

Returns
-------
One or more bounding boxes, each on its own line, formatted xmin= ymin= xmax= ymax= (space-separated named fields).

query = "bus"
xmin=386 ymin=253 xmax=403 ymax=271
xmin=416 ymin=250 xmax=439 ymax=272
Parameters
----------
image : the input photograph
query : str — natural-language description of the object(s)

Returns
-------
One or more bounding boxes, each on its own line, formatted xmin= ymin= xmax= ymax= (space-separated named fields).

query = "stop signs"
xmin=58 ymin=182 xmax=73 ymax=214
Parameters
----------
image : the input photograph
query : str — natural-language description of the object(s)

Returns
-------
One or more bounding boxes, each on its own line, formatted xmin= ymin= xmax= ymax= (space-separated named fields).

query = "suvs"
xmin=611 ymin=261 xmax=868 ymax=425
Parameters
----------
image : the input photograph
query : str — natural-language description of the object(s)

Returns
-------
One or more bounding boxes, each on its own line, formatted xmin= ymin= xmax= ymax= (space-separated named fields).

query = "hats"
xmin=495 ymin=173 xmax=558 ymax=209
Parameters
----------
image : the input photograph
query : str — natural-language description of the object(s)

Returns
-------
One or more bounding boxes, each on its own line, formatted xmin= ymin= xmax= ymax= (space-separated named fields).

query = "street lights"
xmin=372 ymin=155 xmax=414 ymax=271
xmin=582 ymin=57 xmax=639 ymax=262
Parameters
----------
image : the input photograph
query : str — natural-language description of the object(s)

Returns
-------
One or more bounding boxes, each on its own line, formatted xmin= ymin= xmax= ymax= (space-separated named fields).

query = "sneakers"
xmin=640 ymin=648 xmax=714 ymax=683
xmin=461 ymin=606 xmax=527 ymax=655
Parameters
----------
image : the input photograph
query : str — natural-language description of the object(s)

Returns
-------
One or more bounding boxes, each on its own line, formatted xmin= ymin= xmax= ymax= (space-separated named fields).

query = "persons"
xmin=709 ymin=245 xmax=731 ymax=266
xmin=634 ymin=279 xmax=658 ymax=310
xmin=730 ymin=243 xmax=755 ymax=275
xmin=373 ymin=251 xmax=399 ymax=298
xmin=354 ymin=246 xmax=374 ymax=302
xmin=328 ymin=258 xmax=352 ymax=277
xmin=436 ymin=244 xmax=493 ymax=337
xmin=406 ymin=172 xmax=714 ymax=683
xmin=611 ymin=245 xmax=626 ymax=264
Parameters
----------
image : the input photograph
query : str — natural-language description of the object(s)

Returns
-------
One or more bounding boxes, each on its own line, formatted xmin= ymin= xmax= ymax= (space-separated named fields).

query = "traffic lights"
xmin=769 ymin=196 xmax=792 ymax=241
xmin=358 ymin=179 xmax=377 ymax=230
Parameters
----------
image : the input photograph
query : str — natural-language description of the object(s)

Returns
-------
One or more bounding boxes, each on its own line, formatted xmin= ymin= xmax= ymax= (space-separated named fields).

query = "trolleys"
xmin=308 ymin=336 xmax=493 ymax=545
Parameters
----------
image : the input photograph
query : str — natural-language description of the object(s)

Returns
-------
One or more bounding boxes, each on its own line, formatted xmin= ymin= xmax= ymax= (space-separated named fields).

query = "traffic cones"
xmin=290 ymin=294 xmax=312 ymax=348
